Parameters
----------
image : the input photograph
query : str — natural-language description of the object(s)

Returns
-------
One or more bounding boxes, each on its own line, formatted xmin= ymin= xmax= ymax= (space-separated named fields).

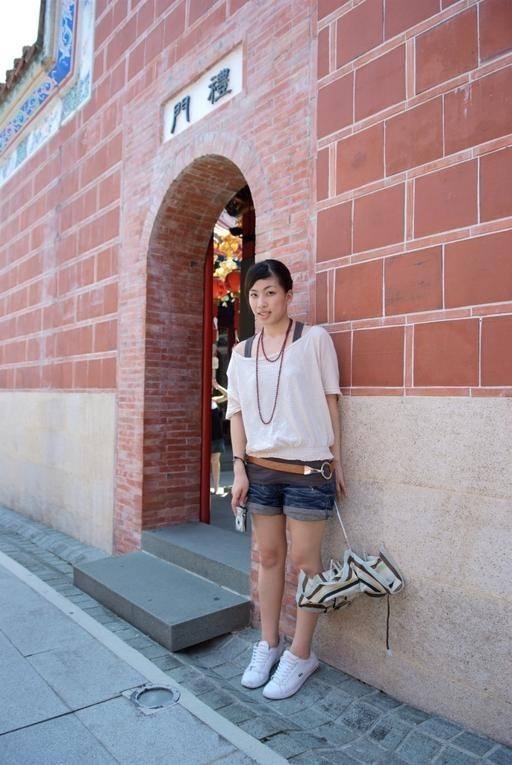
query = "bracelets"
xmin=233 ymin=456 xmax=246 ymax=467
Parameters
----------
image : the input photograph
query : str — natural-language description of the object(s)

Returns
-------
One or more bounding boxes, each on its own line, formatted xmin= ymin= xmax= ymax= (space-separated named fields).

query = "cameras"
xmin=235 ymin=507 xmax=247 ymax=533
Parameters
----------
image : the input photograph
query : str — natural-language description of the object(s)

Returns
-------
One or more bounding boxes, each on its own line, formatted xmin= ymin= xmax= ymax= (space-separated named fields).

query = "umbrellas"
xmin=295 ymin=501 xmax=404 ymax=613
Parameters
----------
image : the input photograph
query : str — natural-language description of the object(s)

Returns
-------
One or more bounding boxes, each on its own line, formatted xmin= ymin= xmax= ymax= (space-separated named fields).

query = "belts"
xmin=246 ymin=456 xmax=337 ymax=480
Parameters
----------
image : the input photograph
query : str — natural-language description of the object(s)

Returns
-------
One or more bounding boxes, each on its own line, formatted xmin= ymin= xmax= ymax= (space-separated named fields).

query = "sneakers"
xmin=241 ymin=633 xmax=319 ymax=700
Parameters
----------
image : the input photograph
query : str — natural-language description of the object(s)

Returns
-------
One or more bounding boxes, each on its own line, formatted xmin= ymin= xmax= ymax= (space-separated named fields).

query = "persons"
xmin=210 ymin=377 xmax=227 ymax=496
xmin=227 ymin=260 xmax=348 ymax=700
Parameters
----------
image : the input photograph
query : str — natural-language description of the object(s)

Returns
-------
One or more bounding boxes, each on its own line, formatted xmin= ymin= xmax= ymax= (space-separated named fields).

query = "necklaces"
xmin=256 ymin=318 xmax=293 ymax=424
